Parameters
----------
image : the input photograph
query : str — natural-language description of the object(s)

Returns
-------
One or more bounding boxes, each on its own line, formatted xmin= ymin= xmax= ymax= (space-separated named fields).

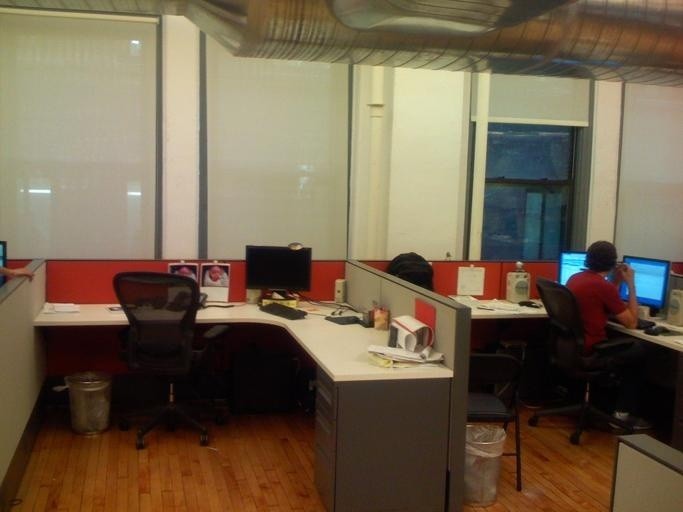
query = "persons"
xmin=0 ymin=265 xmax=34 ymax=283
xmin=202 ymin=265 xmax=227 ymax=287
xmin=174 ymin=267 xmax=197 ymax=282
xmin=564 ymin=240 xmax=655 ymax=432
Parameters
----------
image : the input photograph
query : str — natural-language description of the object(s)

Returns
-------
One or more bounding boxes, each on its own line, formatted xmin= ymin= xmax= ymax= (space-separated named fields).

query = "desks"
xmin=448 ymin=298 xmax=683 ymax=353
xmin=31 ymin=298 xmax=455 ymax=385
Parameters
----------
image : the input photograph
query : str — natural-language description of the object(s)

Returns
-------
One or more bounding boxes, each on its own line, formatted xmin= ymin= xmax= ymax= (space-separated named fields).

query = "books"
xmin=447 ymin=292 xmax=486 ymax=311
xmin=476 ymin=298 xmax=520 ymax=313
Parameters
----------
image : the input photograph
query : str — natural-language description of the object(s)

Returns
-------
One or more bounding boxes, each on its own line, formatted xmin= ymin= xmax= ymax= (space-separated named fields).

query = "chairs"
xmin=111 ymin=269 xmax=231 ymax=451
xmin=467 ymin=278 xmax=634 ymax=492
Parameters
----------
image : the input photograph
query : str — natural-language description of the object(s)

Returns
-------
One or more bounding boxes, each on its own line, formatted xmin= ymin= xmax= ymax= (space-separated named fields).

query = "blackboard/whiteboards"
xmin=0 ymin=3 xmax=165 ymax=259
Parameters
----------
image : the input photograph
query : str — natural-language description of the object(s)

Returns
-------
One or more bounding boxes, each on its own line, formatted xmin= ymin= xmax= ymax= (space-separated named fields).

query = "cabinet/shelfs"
xmin=311 ymin=367 xmax=451 ymax=511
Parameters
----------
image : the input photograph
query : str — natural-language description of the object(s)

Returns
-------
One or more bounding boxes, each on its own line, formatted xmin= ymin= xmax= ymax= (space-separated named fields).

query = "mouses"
xmin=656 ymin=326 xmax=670 ymax=332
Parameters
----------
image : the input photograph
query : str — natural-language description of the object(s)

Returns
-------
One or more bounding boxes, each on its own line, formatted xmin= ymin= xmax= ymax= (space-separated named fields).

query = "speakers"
xmin=335 ymin=279 xmax=346 ymax=303
xmin=666 ymin=289 xmax=683 ymax=327
xmin=506 ymin=272 xmax=531 ymax=303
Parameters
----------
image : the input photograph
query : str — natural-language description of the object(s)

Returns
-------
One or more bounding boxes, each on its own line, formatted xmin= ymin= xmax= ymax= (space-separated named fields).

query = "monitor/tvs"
xmin=245 ymin=245 xmax=311 ymax=299
xmin=619 ymin=255 xmax=670 ymax=316
xmin=0 ymin=241 xmax=6 ymax=288
xmin=559 ymin=251 xmax=591 ymax=286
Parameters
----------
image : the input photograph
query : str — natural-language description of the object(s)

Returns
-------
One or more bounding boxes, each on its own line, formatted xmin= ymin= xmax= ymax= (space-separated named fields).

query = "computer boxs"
xmin=229 ymin=351 xmax=298 ymax=412
xmin=494 ymin=340 xmax=546 ymax=398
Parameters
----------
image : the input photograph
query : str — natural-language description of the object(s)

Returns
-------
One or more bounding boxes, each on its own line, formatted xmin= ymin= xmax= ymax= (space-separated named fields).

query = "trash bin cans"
xmin=64 ymin=369 xmax=115 ymax=435
xmin=461 ymin=422 xmax=507 ymax=507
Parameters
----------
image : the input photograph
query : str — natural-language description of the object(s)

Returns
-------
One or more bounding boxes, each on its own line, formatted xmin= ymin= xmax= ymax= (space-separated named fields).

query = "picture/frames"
xmin=166 ymin=262 xmax=230 ymax=303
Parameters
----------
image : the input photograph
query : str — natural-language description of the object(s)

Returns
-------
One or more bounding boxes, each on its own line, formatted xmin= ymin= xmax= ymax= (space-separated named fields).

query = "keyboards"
xmin=260 ymin=303 xmax=307 ymax=320
xmin=608 ymin=318 xmax=655 ymax=328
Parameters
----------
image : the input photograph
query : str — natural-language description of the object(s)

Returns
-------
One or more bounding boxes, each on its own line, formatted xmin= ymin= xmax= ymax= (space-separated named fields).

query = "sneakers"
xmin=608 ymin=409 xmax=652 ymax=431
